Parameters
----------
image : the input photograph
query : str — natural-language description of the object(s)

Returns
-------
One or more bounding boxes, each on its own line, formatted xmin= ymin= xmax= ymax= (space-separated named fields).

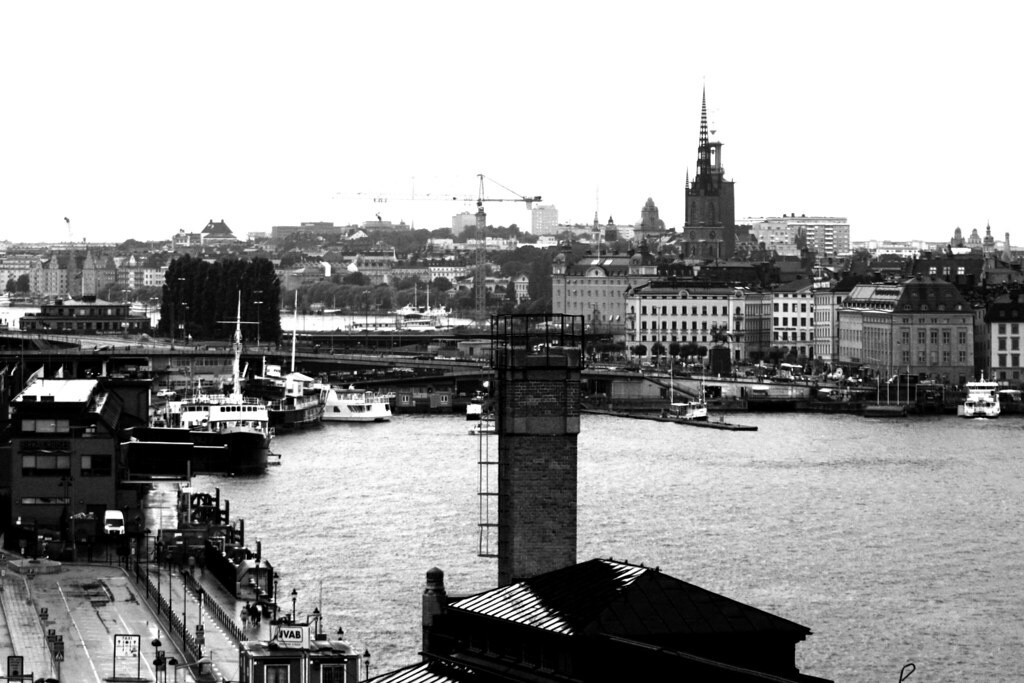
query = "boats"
xmin=667 ymin=402 xmax=708 ymax=421
xmin=163 ymin=289 xmax=271 ymax=450
xmin=963 ymin=369 xmax=1001 ymax=418
xmin=315 ymin=383 xmax=393 ymax=422
xmin=267 ymin=289 xmax=328 ymax=429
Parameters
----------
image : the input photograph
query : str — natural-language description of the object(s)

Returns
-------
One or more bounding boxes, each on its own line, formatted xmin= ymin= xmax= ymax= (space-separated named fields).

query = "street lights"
xmin=151 ymin=639 xmax=162 ymax=683
xmin=337 ymin=627 xmax=345 ymax=640
xmin=313 ymin=607 xmax=320 ymax=640
xmin=291 ymin=589 xmax=298 ymax=624
xmin=272 ymin=573 xmax=280 ymax=621
xmin=152 ymin=656 xmax=178 ymax=683
xmin=174 ymin=656 xmax=213 ymax=683
xmin=363 ymin=649 xmax=371 ymax=681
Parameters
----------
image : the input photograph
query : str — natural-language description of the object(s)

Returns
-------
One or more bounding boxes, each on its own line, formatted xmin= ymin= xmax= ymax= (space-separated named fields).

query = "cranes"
xmin=334 ymin=173 xmax=543 ymax=327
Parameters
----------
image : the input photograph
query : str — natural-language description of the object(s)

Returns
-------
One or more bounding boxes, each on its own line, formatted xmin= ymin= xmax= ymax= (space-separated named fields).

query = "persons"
xmin=240 ymin=601 xmax=261 ymax=627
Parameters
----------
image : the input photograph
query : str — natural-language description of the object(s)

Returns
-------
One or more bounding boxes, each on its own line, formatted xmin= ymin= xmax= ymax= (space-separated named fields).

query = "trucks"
xmin=104 ymin=510 xmax=124 ymax=535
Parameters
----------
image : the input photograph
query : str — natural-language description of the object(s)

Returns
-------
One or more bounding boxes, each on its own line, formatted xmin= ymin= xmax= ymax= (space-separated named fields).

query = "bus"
xmin=781 ymin=362 xmax=804 ymax=381
